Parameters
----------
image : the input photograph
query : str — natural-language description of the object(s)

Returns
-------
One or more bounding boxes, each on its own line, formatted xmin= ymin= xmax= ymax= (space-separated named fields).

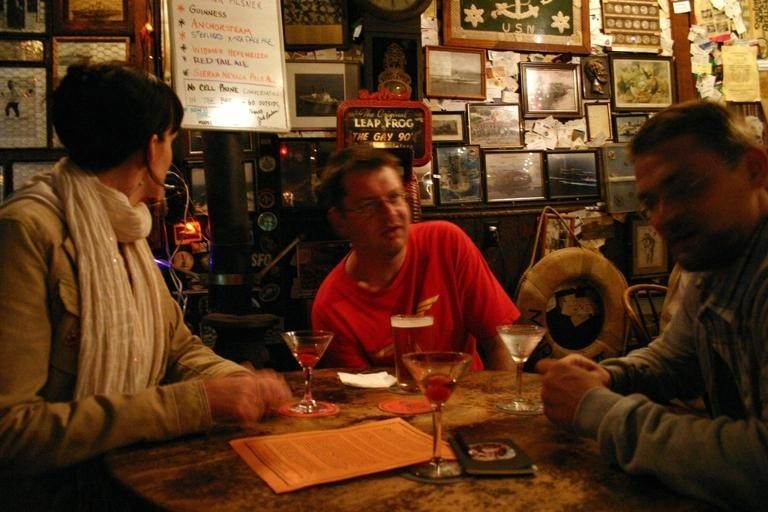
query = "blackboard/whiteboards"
xmin=167 ymin=0 xmax=291 ymax=135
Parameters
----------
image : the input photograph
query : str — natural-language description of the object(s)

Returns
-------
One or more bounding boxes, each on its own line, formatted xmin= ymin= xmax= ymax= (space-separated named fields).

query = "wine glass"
xmin=280 ymin=327 xmax=335 ymax=416
xmin=492 ymin=322 xmax=551 ymax=414
xmin=398 ymin=348 xmax=473 ymax=486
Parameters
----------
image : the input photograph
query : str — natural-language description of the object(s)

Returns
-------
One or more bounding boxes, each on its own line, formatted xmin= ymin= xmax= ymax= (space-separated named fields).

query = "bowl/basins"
xmin=296 ymin=94 xmax=337 ymax=116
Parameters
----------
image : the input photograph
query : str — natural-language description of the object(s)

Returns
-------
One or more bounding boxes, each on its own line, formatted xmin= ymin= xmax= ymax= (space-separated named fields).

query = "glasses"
xmin=347 ymin=192 xmax=408 ymax=217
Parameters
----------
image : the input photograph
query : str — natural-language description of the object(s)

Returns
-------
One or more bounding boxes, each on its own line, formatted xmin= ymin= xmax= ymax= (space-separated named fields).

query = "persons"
xmin=5 ymin=79 xmax=22 ymax=120
xmin=537 ymin=99 xmax=768 ymax=509
xmin=313 ymin=144 xmax=521 ymax=375
xmin=586 ymin=59 xmax=608 ymax=96
xmin=3 ymin=60 xmax=292 ymax=497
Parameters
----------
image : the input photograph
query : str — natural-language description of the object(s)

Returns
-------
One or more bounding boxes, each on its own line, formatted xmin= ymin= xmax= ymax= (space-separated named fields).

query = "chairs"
xmin=621 ymin=281 xmax=671 ymax=356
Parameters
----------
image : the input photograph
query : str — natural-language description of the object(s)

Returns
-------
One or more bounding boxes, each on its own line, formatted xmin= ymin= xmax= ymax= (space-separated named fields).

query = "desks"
xmin=106 ymin=367 xmax=636 ymax=512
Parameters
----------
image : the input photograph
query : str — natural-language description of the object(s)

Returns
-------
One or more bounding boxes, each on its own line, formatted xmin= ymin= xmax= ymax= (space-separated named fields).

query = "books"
xmin=449 ymin=433 xmax=538 ymax=482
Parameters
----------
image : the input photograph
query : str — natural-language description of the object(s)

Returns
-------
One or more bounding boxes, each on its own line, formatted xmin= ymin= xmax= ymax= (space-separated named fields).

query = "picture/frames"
xmin=1 ymin=1 xmax=130 ymax=150
xmin=283 ymin=62 xmax=357 ymax=133
xmin=442 ymin=0 xmax=592 ymax=52
xmin=423 ymin=45 xmax=485 ymax=102
xmin=605 ymin=51 xmax=676 ymax=110
xmin=519 ymin=63 xmax=582 ymax=118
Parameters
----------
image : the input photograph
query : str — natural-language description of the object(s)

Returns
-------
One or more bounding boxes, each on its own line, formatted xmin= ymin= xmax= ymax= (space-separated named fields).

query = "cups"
xmin=388 ymin=313 xmax=434 ymax=395
